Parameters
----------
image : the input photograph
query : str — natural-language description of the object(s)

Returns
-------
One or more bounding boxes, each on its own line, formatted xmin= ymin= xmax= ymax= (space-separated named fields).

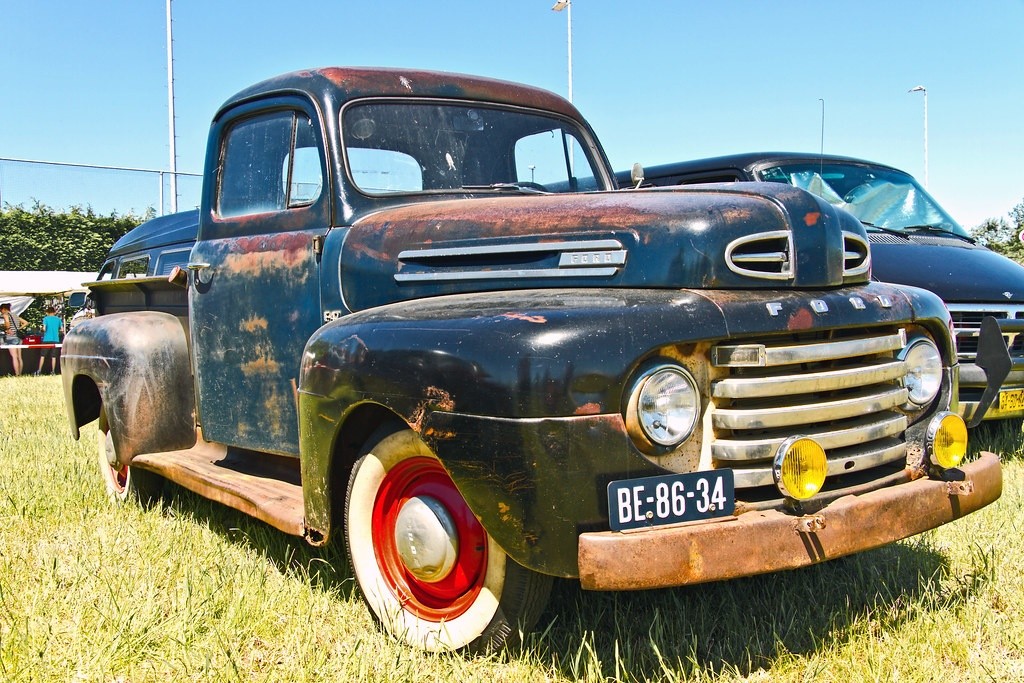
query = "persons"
xmin=0 ymin=303 xmax=28 ymax=377
xmin=31 ymin=307 xmax=63 ymax=376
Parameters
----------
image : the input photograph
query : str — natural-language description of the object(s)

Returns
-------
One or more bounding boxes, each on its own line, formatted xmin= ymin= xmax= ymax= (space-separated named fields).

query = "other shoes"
xmin=51 ymin=371 xmax=55 ymax=375
xmin=33 ymin=371 xmax=41 ymax=375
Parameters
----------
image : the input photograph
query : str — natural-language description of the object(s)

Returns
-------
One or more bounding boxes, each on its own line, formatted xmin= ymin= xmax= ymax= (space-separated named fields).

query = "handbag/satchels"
xmin=16 ymin=330 xmax=27 ymax=339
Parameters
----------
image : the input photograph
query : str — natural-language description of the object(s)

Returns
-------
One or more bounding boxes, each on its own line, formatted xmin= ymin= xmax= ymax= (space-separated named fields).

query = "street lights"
xmin=528 ymin=166 xmax=536 ymax=183
xmin=909 ymin=86 xmax=930 ymax=193
xmin=553 ymin=0 xmax=574 ymax=176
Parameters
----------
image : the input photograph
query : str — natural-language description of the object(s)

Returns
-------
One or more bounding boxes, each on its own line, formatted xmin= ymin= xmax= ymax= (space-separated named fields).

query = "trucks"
xmin=61 ymin=63 xmax=1014 ymax=663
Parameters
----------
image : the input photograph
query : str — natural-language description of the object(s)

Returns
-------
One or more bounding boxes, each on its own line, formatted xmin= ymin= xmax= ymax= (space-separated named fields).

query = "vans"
xmin=543 ymin=150 xmax=1024 ymax=429
xmin=68 ymin=199 xmax=315 ymax=329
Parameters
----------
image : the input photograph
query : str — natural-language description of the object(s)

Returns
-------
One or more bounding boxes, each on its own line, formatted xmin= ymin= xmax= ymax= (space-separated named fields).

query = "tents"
xmin=0 ymin=270 xmax=147 ymax=323
